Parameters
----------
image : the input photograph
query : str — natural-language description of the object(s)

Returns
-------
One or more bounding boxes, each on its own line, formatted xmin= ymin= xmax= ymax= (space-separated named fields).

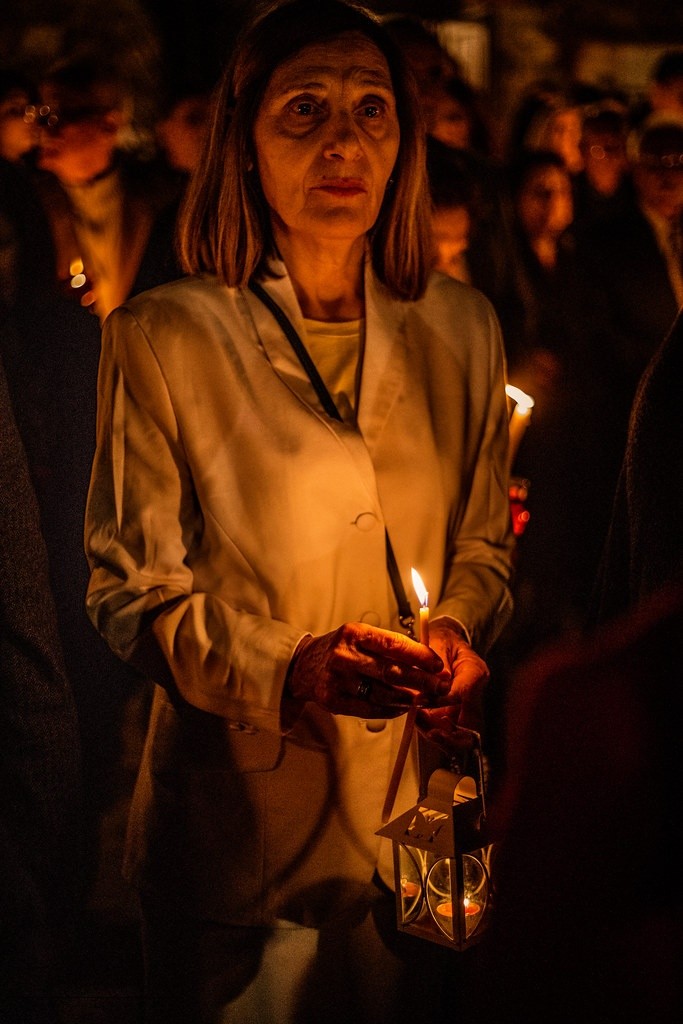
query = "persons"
xmin=363 ymin=0 xmax=683 ymax=673
xmin=85 ymin=0 xmax=515 ymax=1024
xmin=1 ymin=356 xmax=85 ymax=1023
xmin=1 ymin=2 xmax=235 ymax=767
xmin=466 ymin=308 xmax=683 ymax=1024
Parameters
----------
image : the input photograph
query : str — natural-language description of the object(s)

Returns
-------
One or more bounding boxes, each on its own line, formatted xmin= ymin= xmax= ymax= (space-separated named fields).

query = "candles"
xmin=382 ymin=599 xmax=428 ymax=823
xmin=507 ymin=405 xmax=530 ymax=465
xmin=436 ymin=901 xmax=481 ymax=935
xmin=404 ymin=883 xmax=422 ymax=908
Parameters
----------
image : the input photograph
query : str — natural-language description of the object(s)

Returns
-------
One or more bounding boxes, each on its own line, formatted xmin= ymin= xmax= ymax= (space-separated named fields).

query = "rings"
xmin=356 ymin=676 xmax=372 ymax=700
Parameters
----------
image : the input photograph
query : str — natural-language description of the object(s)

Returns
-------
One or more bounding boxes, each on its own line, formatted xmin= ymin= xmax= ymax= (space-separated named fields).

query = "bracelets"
xmin=429 ymin=617 xmax=465 ymax=637
